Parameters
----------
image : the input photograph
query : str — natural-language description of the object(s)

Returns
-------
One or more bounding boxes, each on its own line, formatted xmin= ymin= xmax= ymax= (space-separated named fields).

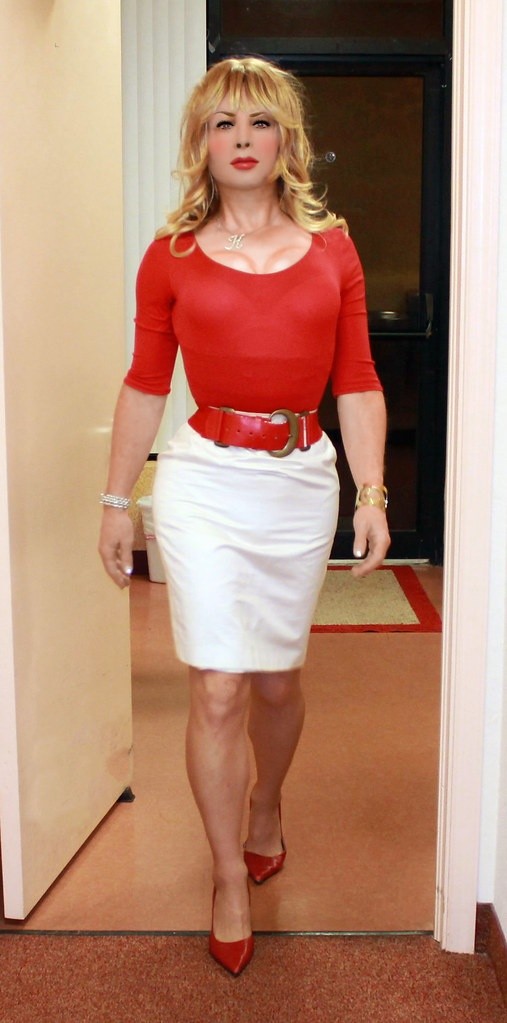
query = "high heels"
xmin=209 ymin=877 xmax=254 ymax=976
xmin=243 ymin=792 xmax=287 ymax=885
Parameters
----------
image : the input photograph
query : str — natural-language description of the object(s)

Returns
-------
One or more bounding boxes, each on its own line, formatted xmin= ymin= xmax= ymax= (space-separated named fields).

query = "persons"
xmin=98 ymin=55 xmax=392 ymax=980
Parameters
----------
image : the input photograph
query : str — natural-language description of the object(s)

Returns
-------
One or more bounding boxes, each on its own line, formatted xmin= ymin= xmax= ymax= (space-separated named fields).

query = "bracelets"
xmin=352 ymin=481 xmax=391 ymax=509
xmin=99 ymin=494 xmax=131 ymax=510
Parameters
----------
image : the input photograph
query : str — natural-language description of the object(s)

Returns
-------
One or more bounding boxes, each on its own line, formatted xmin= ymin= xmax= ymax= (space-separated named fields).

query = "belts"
xmin=189 ymin=405 xmax=321 ymax=458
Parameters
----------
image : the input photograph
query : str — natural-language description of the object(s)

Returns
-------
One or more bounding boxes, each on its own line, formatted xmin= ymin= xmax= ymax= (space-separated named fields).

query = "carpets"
xmin=310 ymin=563 xmax=443 ymax=634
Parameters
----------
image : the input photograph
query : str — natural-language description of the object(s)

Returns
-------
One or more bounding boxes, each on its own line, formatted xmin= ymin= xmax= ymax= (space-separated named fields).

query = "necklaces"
xmin=219 ymin=224 xmax=265 ymax=253
